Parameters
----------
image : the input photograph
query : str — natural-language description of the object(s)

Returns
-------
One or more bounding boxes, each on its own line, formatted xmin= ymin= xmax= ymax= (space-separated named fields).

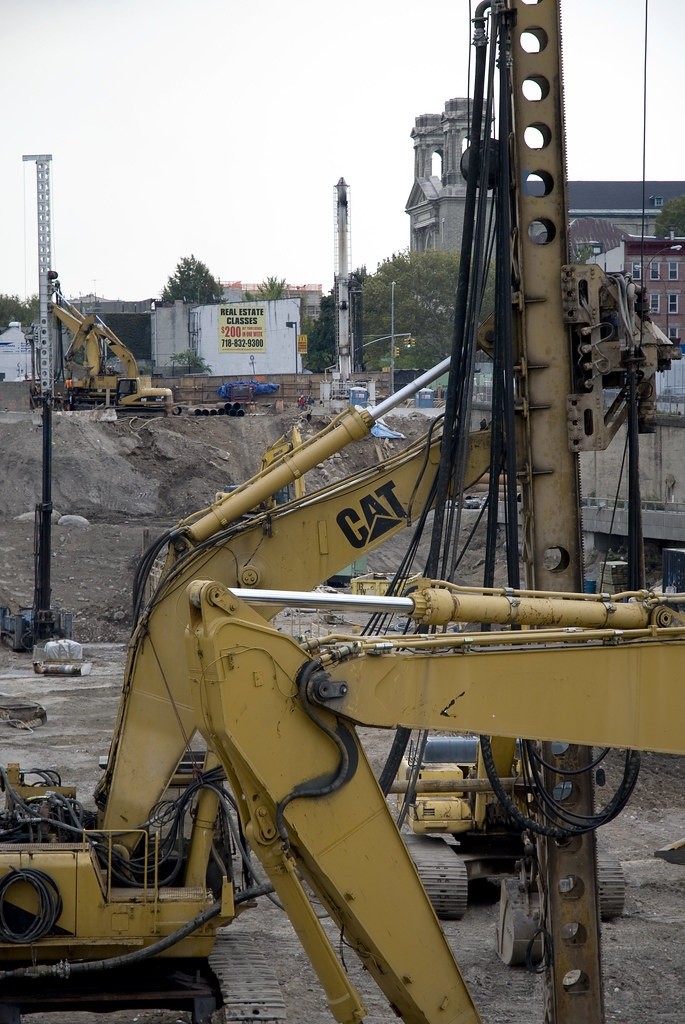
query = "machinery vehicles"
xmin=21 ymin=154 xmax=174 ymax=417
xmin=0 ymin=400 xmax=72 ymax=654
xmin=0 ymin=0 xmax=685 ymax=1024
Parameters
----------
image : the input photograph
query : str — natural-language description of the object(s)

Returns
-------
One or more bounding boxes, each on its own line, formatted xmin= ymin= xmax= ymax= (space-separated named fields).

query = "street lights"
xmin=286 ymin=322 xmax=298 ymax=374
xmin=391 ymin=282 xmax=396 ymax=395
xmin=634 ymin=264 xmax=669 ymax=338
xmin=645 ymin=244 xmax=682 ymax=288
